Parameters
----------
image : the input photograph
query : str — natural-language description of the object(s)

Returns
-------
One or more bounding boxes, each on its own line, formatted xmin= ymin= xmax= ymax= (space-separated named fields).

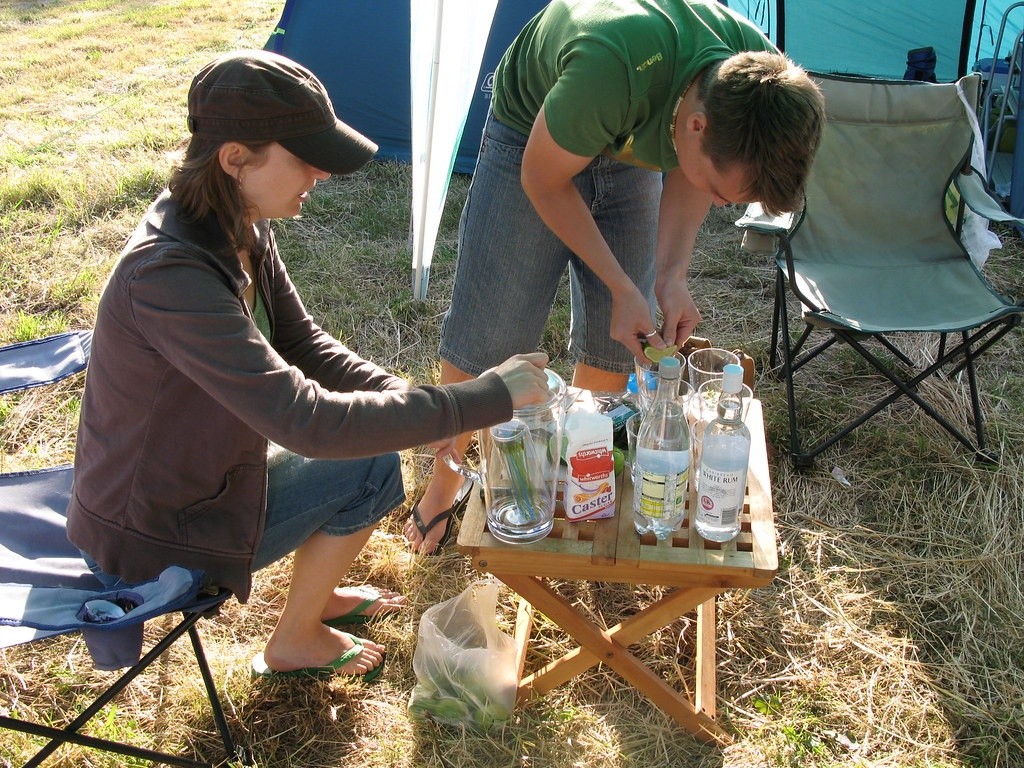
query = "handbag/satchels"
xmin=408 ymin=580 xmax=518 ymax=735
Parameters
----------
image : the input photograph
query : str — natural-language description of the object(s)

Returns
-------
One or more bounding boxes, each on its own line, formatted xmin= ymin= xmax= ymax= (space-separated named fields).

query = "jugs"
xmin=443 ymin=366 xmax=567 ymax=545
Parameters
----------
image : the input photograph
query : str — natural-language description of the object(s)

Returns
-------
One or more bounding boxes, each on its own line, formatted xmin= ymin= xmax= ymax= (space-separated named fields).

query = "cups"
xmin=626 ymin=411 xmax=647 ymax=486
xmin=687 ymin=348 xmax=740 ymax=396
xmin=634 ymin=351 xmax=686 ymax=412
xmin=698 ymin=379 xmax=754 ymax=424
xmin=691 ymin=420 xmax=716 ymax=491
xmin=638 ymin=377 xmax=695 ymax=428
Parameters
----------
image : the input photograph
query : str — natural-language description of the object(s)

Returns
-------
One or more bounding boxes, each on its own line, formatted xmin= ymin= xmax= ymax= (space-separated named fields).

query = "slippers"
xmin=404 ymin=476 xmax=473 ymax=554
xmin=254 ymin=583 xmax=407 ymax=684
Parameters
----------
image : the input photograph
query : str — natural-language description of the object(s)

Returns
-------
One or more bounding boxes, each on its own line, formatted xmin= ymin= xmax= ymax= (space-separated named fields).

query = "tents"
xmin=264 ymin=0 xmax=1024 ymax=184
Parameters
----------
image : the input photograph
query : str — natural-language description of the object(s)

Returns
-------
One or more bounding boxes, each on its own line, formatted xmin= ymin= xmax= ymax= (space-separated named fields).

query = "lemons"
xmin=615 ymin=447 xmax=624 ymax=478
xmin=408 ymin=671 xmax=508 ymax=732
xmin=549 ymin=434 xmax=568 ymax=467
xmin=643 ymin=340 xmax=678 ymax=363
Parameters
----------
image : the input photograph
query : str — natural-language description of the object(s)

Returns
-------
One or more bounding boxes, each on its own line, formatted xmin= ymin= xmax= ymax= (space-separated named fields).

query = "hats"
xmin=186 ymin=49 xmax=380 ymax=174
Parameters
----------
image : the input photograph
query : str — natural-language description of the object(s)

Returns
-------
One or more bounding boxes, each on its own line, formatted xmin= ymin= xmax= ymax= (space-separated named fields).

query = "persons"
xmin=401 ymin=0 xmax=828 ymax=557
xmin=63 ymin=51 xmax=550 ymax=680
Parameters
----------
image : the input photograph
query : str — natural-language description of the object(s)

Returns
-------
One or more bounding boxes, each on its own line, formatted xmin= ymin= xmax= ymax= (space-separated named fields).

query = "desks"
xmin=455 ymin=399 xmax=779 ymax=743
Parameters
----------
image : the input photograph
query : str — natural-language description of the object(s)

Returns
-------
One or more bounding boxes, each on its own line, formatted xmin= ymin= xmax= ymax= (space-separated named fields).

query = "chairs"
xmin=0 ymin=328 xmax=263 ymax=768
xmin=735 ymin=2 xmax=1024 ymax=476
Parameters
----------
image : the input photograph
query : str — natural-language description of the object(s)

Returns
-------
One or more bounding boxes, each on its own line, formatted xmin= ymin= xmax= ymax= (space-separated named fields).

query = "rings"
xmin=646 ymin=330 xmax=658 ymax=337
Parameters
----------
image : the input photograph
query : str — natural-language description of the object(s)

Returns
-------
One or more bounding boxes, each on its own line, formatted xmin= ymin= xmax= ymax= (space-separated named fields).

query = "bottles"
xmin=694 ymin=364 xmax=751 ymax=543
xmin=632 ymin=357 xmax=691 ymax=543
